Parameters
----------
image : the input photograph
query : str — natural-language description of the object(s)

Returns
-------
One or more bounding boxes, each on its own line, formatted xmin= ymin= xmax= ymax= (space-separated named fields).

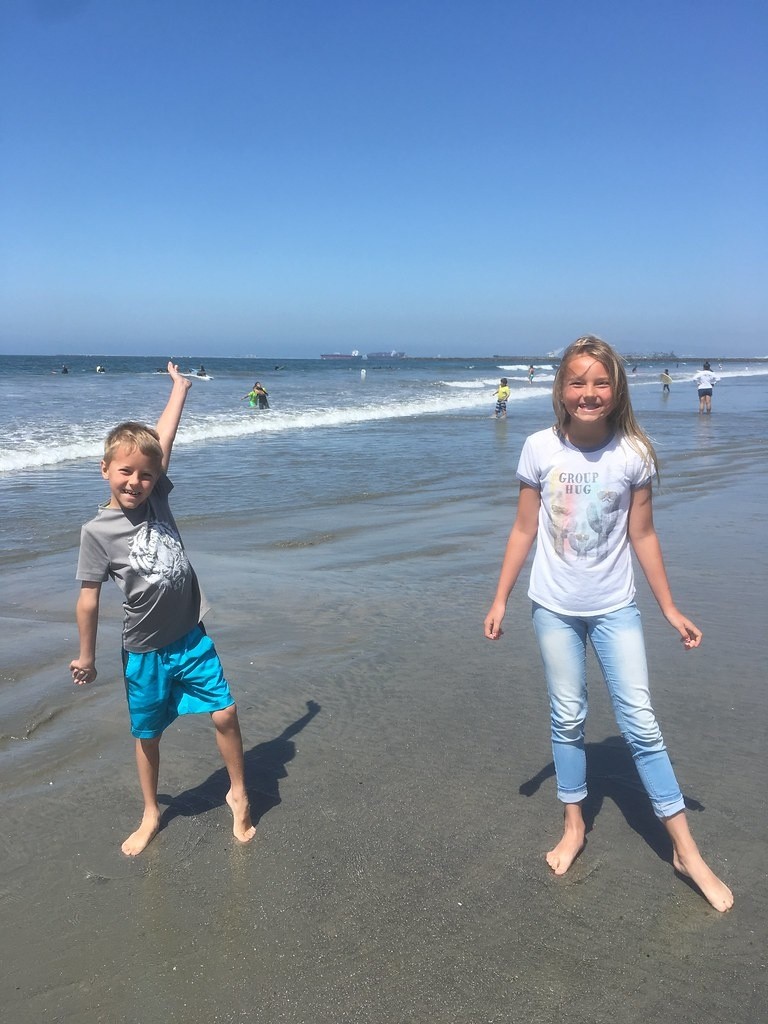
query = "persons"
xmin=493 ymin=377 xmax=510 ymax=419
xmin=528 ymin=362 xmax=722 ymax=416
xmin=482 ymin=334 xmax=736 ymax=914
xmin=241 ymin=381 xmax=271 ymax=410
xmin=68 ymin=362 xmax=255 ymax=858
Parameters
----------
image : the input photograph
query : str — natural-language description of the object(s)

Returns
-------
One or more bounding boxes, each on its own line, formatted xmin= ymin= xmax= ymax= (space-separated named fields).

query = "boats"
xmin=367 ymin=350 xmax=406 ymax=360
xmin=320 ymin=352 xmax=363 ymax=361
